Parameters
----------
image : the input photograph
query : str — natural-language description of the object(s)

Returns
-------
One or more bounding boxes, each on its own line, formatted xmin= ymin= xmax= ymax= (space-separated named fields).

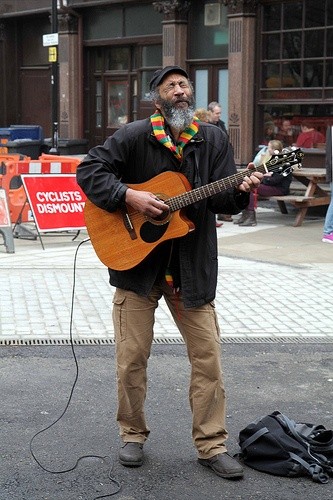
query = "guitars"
xmin=83 ymin=146 xmax=305 ymax=270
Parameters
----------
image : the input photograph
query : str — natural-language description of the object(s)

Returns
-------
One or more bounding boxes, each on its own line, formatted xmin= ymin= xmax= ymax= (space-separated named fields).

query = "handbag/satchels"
xmin=237 ymin=407 xmax=332 ymax=485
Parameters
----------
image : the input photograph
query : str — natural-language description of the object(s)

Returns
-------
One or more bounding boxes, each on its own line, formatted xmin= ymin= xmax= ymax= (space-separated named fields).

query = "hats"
xmin=148 ymin=65 xmax=190 ymax=89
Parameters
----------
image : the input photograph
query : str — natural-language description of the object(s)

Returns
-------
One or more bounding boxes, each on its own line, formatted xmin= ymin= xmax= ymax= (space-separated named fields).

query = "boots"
xmin=240 ymin=211 xmax=258 ymax=226
xmin=234 ymin=211 xmax=250 ymax=224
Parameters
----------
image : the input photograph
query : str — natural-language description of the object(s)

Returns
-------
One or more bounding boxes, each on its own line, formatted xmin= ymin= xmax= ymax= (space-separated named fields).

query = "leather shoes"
xmin=196 ymin=452 xmax=243 ymax=480
xmin=120 ymin=440 xmax=145 ymax=466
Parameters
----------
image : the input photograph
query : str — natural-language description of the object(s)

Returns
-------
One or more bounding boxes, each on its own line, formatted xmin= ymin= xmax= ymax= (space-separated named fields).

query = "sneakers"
xmin=321 ymin=231 xmax=333 ymax=243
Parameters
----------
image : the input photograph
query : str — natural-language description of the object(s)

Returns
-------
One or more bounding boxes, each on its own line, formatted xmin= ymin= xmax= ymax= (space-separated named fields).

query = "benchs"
xmin=257 ymin=195 xmax=315 ymax=227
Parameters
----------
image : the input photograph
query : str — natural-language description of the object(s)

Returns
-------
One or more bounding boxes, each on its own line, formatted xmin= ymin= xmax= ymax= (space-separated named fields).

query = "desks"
xmin=291 ymin=167 xmax=332 ymax=227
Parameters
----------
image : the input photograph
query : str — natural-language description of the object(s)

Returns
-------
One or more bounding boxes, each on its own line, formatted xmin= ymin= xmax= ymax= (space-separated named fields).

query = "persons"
xmin=273 ymin=118 xmax=295 ymax=147
xmin=321 ymin=123 xmax=333 ymax=245
xmin=233 ymin=140 xmax=293 ymax=227
xmin=74 ymin=65 xmax=265 ymax=481
xmin=259 ymin=121 xmax=277 ymax=147
xmin=294 ymin=118 xmax=326 ymax=149
